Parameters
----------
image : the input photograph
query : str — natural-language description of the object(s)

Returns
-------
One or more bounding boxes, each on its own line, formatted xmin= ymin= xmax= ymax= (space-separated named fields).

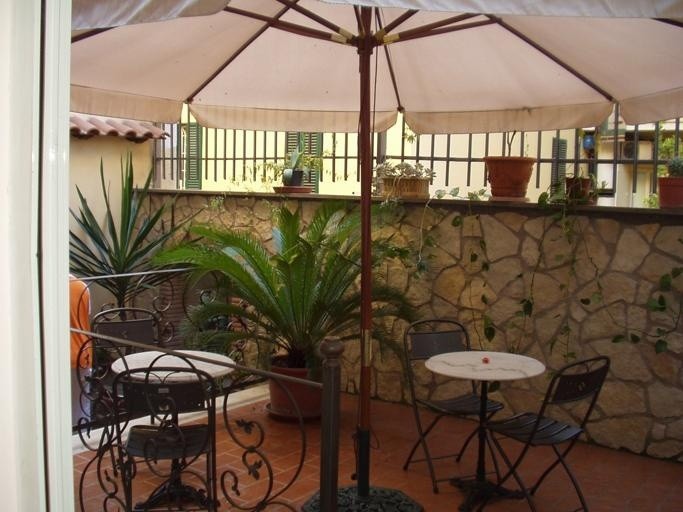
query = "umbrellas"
xmin=67 ymin=2 xmax=683 ymax=497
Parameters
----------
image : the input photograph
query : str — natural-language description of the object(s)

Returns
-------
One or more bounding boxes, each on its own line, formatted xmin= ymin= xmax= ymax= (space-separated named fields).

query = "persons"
xmin=67 ymin=271 xmax=93 ymax=425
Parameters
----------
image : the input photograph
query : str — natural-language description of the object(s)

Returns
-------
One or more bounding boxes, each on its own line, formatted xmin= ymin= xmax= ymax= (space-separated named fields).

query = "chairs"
xmin=401 ymin=318 xmax=502 ymax=495
xmin=473 ymin=355 xmax=610 ymax=512
xmin=90 ymin=308 xmax=219 ymax=512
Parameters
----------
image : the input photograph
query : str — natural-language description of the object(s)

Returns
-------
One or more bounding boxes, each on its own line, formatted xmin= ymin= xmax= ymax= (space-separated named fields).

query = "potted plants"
xmin=537 ymin=169 xmax=609 ymax=245
xmin=153 ymin=204 xmax=427 ymax=423
xmin=206 ymin=133 xmax=348 ymax=228
xmin=369 ymin=161 xmax=436 ymax=210
xmin=657 ymin=155 xmax=682 ymax=210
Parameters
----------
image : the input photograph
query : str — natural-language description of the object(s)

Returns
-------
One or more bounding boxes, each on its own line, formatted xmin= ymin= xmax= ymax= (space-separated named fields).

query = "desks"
xmin=423 ymin=350 xmax=546 ymax=511
xmin=110 ymin=350 xmax=235 ymax=510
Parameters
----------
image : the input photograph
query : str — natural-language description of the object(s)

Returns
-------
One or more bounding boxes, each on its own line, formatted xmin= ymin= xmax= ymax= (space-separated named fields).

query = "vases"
xmin=483 ymin=155 xmax=534 ymax=200
